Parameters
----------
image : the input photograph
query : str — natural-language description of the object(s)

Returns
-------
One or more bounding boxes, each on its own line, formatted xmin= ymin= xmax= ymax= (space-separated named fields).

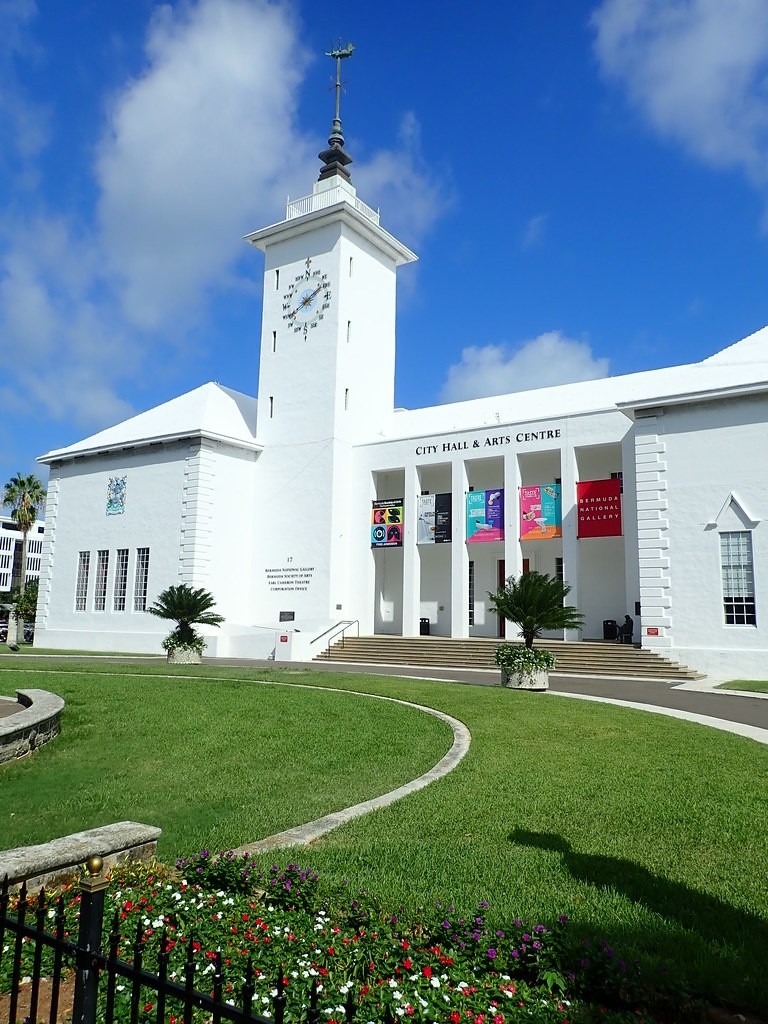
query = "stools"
xmin=621 ymin=633 xmax=633 ymax=643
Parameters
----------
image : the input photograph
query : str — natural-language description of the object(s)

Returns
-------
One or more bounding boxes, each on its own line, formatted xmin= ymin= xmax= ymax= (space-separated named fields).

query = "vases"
xmin=500 ymin=668 xmax=548 ymax=690
xmin=167 ymin=648 xmax=203 ymax=664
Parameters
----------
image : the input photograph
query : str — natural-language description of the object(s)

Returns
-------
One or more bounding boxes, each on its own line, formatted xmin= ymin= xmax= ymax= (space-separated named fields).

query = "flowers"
xmin=147 ymin=582 xmax=225 ymax=656
xmin=486 ymin=569 xmax=587 ymax=674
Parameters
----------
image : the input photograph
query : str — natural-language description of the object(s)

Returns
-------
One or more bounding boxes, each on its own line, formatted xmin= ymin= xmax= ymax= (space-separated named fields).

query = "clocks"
xmin=282 ymin=269 xmax=331 ymax=341
